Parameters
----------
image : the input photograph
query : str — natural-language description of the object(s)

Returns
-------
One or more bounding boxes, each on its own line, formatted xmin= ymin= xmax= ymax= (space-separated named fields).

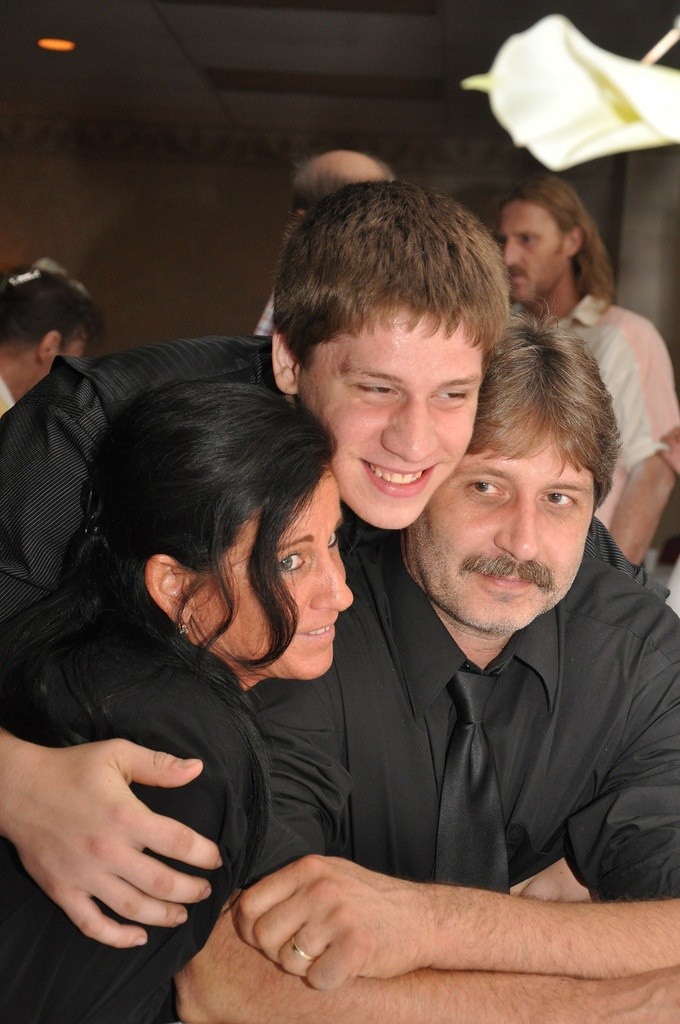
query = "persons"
xmin=191 ymin=315 xmax=679 ymax=1024
xmin=2 ymin=377 xmax=354 ymax=1023
xmin=1 ymin=182 xmax=509 ymax=1024
xmin=0 ymin=149 xmax=680 ymax=565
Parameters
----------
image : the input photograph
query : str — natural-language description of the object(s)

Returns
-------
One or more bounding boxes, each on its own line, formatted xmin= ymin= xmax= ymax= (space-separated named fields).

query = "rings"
xmin=290 ymin=936 xmax=312 ymax=963
